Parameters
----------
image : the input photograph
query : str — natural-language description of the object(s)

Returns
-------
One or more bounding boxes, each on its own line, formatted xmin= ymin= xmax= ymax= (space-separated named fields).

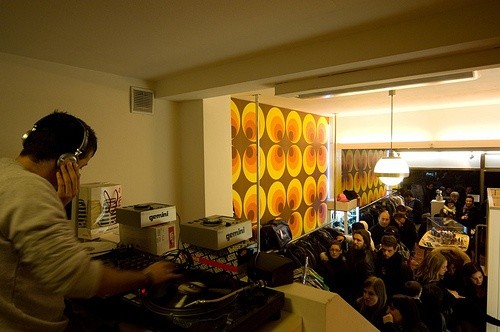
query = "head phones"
xmin=22 ymin=113 xmax=89 ymax=169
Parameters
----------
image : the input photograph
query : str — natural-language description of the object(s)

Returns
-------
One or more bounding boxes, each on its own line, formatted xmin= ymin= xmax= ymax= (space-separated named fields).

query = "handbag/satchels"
xmin=260 ymin=218 xmax=292 ymax=251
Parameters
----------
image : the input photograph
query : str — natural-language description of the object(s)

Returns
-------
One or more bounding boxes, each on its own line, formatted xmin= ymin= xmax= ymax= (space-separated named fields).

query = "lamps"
xmin=374 ymin=90 xmax=409 ymax=185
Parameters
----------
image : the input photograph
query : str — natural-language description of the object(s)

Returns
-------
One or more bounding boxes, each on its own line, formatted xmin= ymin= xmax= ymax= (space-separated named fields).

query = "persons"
xmin=317 ymin=179 xmax=487 ymax=331
xmin=0 ymin=110 xmax=176 ymax=332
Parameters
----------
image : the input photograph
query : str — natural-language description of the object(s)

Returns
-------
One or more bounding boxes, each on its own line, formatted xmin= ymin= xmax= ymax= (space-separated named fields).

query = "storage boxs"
xmin=116 ymin=202 xmax=252 ymax=257
